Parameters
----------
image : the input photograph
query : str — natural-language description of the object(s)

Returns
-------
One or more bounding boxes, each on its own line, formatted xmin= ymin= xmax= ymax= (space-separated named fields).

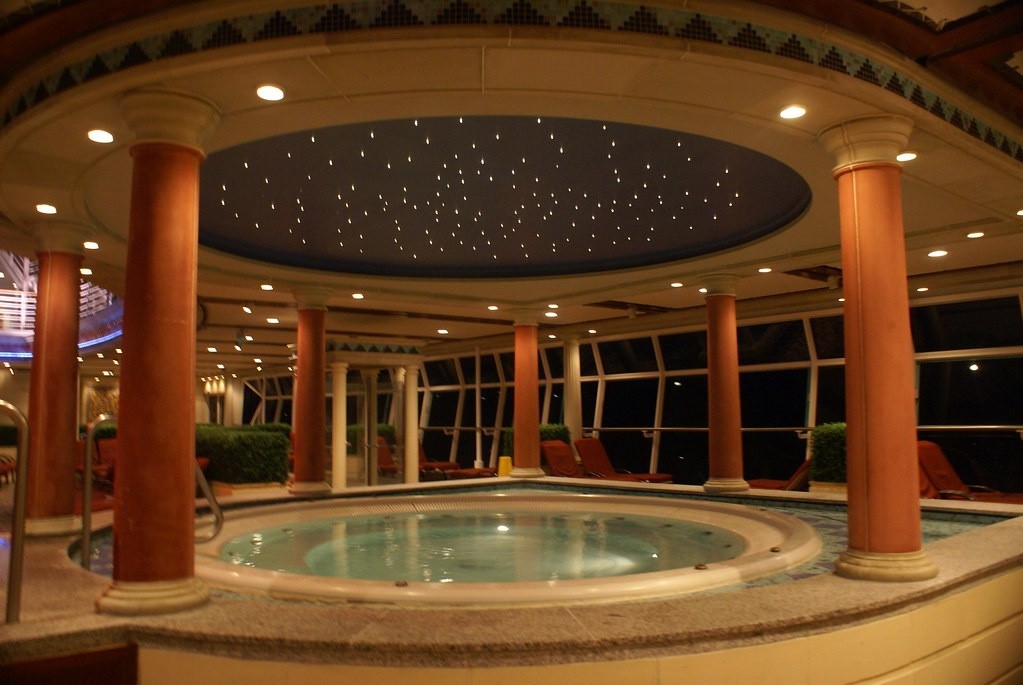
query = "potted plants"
xmin=808 ymin=421 xmax=847 ymax=494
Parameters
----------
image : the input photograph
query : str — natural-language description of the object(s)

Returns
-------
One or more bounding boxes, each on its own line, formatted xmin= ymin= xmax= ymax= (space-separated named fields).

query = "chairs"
xmin=916 ymin=440 xmax=1023 ymax=506
xmin=748 ymin=454 xmax=815 ymax=492
xmin=376 ymin=436 xmax=497 ymax=477
xmin=540 ymin=438 xmax=671 ymax=485
xmin=70 ymin=434 xmax=209 ymax=516
